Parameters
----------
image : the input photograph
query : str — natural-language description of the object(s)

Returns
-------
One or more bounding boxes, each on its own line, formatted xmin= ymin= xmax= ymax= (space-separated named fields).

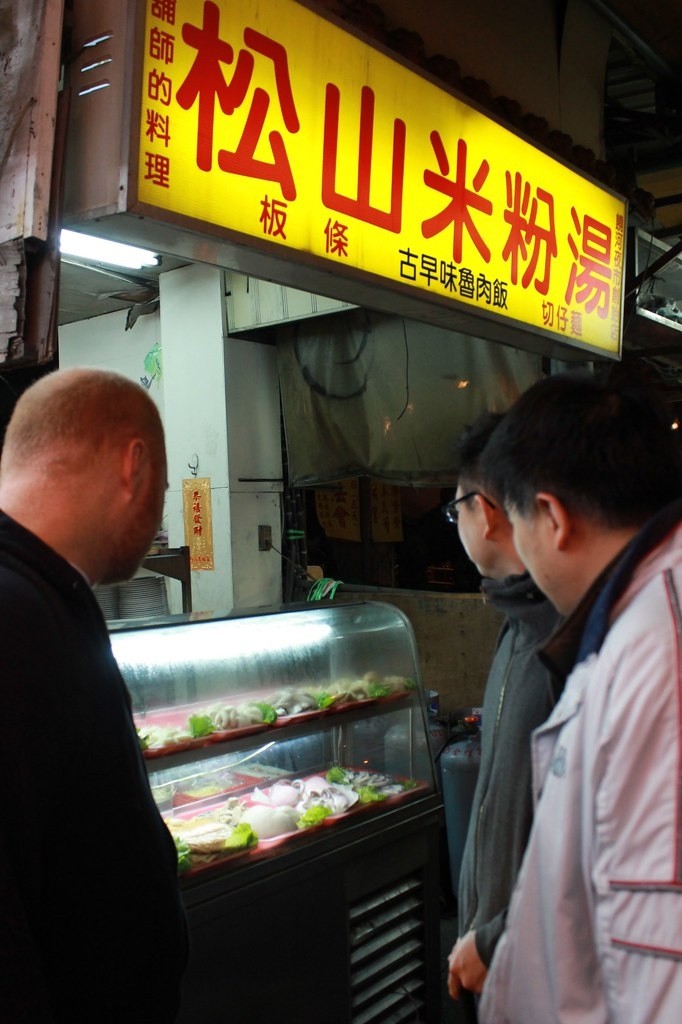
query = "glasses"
xmin=441 ymin=490 xmax=496 ymax=523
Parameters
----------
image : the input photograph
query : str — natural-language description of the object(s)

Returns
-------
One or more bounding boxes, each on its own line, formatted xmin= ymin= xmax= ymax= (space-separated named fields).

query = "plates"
xmin=211 ymin=724 xmax=268 ymax=733
xmin=90 ymin=574 xmax=170 ymax=624
xmin=296 ymin=791 xmax=358 ymax=814
xmin=276 ymin=707 xmax=329 ymax=719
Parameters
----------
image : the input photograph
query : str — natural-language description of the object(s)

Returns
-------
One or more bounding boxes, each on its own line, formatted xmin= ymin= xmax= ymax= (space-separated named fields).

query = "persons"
xmin=446 ymin=409 xmax=561 ymax=1024
xmin=479 ymin=369 xmax=682 ymax=1024
xmin=0 ymin=369 xmax=188 ymax=1024
